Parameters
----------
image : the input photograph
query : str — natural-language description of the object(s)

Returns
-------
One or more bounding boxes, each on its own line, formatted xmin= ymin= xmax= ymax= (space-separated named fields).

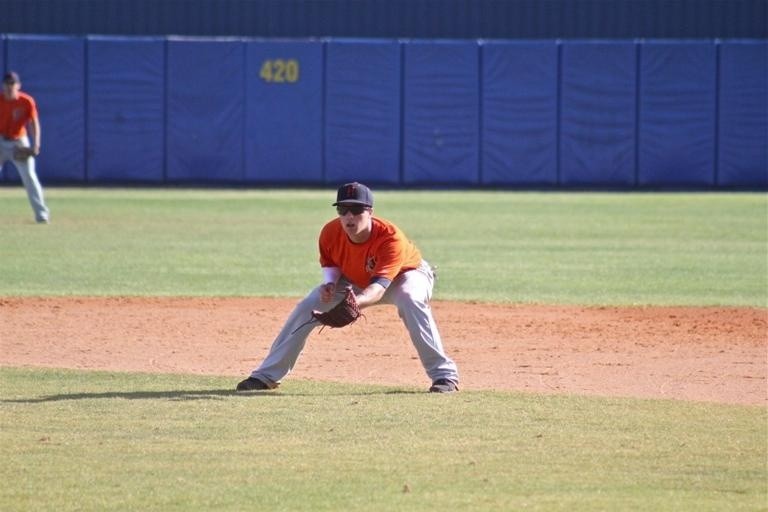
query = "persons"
xmin=0 ymin=68 xmax=50 ymax=227
xmin=232 ymin=180 xmax=461 ymax=396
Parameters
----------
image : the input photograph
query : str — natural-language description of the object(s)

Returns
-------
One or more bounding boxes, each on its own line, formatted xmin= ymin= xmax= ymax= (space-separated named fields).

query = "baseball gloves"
xmin=310 ymin=284 xmax=361 ymax=327
xmin=10 ymin=144 xmax=34 ymax=162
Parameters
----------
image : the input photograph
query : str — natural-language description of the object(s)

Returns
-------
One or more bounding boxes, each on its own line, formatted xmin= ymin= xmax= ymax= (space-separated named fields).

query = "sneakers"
xmin=235 ymin=374 xmax=274 ymax=391
xmin=428 ymin=377 xmax=460 ymax=392
xmin=36 ymin=215 xmax=49 ymax=224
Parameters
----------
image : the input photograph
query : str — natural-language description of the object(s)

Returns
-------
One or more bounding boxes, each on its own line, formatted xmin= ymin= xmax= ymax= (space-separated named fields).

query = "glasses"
xmin=336 ymin=205 xmax=369 ymax=215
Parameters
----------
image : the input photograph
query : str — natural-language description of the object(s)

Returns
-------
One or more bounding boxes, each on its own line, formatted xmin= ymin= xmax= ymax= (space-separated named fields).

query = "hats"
xmin=333 ymin=182 xmax=374 ymax=207
xmin=1 ymin=70 xmax=21 ymax=83
